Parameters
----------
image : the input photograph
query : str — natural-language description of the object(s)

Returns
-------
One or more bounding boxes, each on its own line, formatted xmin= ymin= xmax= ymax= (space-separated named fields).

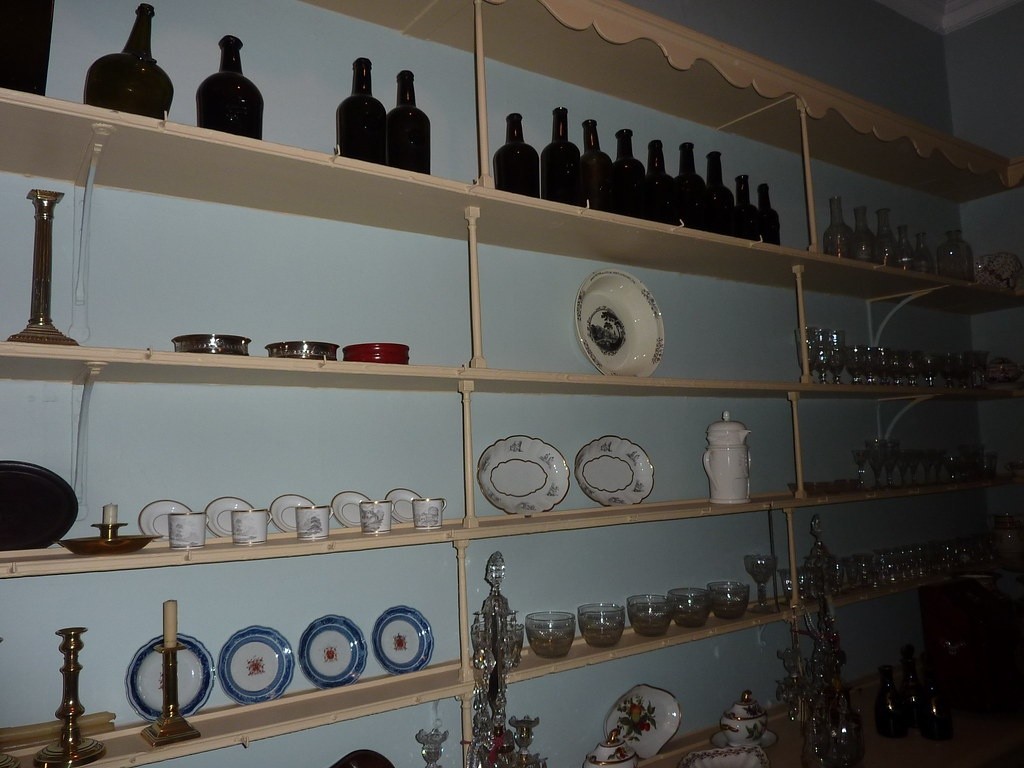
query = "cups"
xmin=359 ymin=500 xmax=395 ymax=534
xmin=412 ymin=498 xmax=447 ymax=529
xmin=231 ymin=509 xmax=273 ymax=545
xmin=294 ymin=505 xmax=334 ymax=540
xmin=169 ymin=512 xmax=209 ymax=549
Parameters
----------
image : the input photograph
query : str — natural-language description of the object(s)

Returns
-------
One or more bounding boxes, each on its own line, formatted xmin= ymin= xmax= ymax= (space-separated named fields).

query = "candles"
xmin=104 ymin=503 xmax=118 ymax=524
xmin=163 ymin=599 xmax=177 ymax=648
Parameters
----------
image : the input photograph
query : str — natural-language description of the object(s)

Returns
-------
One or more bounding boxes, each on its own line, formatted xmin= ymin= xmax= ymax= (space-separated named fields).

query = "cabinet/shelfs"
xmin=0 ymin=0 xmax=1023 ymax=768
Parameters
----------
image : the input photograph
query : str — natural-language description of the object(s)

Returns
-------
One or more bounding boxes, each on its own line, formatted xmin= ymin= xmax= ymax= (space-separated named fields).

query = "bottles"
xmin=84 ymin=3 xmax=174 ymax=120
xmin=196 ymin=35 xmax=264 ymax=141
xmin=873 ymin=643 xmax=955 ymax=742
xmin=494 ymin=107 xmax=782 ymax=244
xmin=336 ymin=57 xmax=431 ymax=176
xmin=823 ymin=197 xmax=974 ymax=282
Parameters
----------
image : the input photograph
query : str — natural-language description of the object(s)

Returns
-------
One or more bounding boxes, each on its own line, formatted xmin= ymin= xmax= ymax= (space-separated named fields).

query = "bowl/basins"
xmin=836 ymin=479 xmax=857 ymax=491
xmin=525 ymin=582 xmax=750 ymax=659
xmin=787 ymin=481 xmax=843 ymax=497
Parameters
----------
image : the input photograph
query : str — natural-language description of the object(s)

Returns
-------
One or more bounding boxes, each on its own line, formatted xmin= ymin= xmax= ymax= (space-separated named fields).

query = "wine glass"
xmin=777 ymin=533 xmax=994 ymax=605
xmin=745 ymin=553 xmax=778 ymax=614
xmin=794 ymin=326 xmax=948 ymax=387
xmin=852 ymin=436 xmax=924 ymax=492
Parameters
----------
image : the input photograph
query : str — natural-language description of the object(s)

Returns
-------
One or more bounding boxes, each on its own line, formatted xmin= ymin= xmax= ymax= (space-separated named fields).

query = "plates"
xmin=126 ymin=605 xmax=435 ymax=722
xmin=138 ymin=488 xmax=421 ymax=542
xmin=477 ymin=435 xmax=570 ymax=514
xmin=604 ymin=681 xmax=682 ymax=759
xmin=573 ymin=435 xmax=654 ymax=507
xmin=575 ymin=269 xmax=665 ymax=377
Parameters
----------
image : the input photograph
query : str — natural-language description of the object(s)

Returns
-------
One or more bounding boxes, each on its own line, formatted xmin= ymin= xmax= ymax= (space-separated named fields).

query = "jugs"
xmin=703 ymin=410 xmax=752 ymax=504
